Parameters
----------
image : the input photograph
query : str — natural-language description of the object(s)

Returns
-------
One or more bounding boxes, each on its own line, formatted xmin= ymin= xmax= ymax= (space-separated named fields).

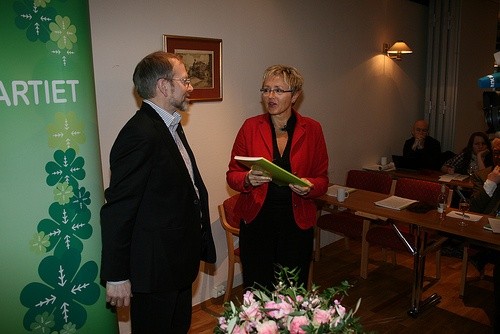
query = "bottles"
xmin=437 ymin=185 xmax=447 ymax=222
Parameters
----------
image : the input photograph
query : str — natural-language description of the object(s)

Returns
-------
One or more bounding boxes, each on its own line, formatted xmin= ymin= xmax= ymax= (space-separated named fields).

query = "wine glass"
xmin=457 ymin=199 xmax=470 ymax=226
xmin=466 ymin=165 xmax=475 ymax=184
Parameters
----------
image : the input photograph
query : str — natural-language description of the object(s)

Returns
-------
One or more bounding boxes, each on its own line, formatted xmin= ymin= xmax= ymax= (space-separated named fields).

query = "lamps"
xmin=383 ymin=40 xmax=413 ymax=61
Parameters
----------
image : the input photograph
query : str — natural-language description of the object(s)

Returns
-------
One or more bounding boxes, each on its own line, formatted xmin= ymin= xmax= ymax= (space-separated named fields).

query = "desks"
xmin=317 ymin=169 xmax=500 ymax=316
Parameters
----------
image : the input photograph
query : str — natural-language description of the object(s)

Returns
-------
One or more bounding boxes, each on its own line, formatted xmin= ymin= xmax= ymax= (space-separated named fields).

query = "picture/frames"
xmin=162 ymin=34 xmax=224 ymax=103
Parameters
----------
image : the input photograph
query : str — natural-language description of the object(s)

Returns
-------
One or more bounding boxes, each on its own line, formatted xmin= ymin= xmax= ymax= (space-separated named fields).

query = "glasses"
xmin=157 ymin=75 xmax=191 ymax=84
xmin=260 ymin=87 xmax=292 ymax=96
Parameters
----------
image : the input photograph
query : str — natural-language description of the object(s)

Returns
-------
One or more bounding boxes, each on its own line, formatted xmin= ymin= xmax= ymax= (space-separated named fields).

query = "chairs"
xmin=219 ymin=169 xmax=453 ymax=305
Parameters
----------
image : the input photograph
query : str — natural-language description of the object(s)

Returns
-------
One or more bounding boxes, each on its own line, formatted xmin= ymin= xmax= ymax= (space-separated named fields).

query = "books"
xmin=375 ymin=196 xmax=417 ymax=210
xmin=234 ymin=156 xmax=309 ymax=187
xmin=326 ymin=184 xmax=357 ymax=197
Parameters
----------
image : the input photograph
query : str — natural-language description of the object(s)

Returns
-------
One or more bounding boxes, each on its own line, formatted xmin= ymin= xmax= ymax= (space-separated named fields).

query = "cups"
xmin=381 ymin=157 xmax=387 ymax=165
xmin=448 ymin=167 xmax=454 ymax=174
xmin=337 ymin=188 xmax=349 ymax=202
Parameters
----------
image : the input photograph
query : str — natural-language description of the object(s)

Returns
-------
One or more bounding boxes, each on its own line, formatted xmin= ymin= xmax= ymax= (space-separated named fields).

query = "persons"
xmin=403 ymin=120 xmax=442 ymax=172
xmin=226 ymin=64 xmax=329 ymax=301
xmin=443 ymin=132 xmax=500 ymax=214
xmin=101 ymin=51 xmax=217 ymax=334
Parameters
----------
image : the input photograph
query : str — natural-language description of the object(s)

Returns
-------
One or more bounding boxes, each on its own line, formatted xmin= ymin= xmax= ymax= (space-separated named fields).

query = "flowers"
xmin=215 ymin=263 xmax=367 ymax=334
